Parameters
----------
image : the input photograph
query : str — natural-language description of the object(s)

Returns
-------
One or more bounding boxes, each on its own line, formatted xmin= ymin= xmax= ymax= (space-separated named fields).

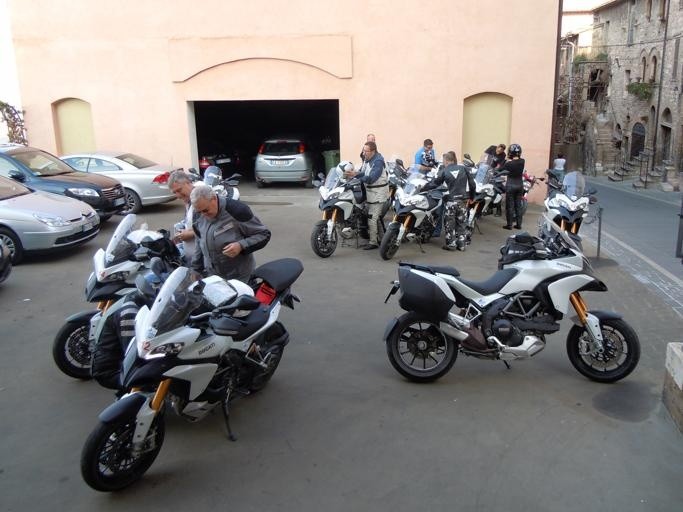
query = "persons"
xmin=167 ymin=171 xmax=196 ymax=267
xmin=346 ymin=133 xmax=524 ymax=252
xmin=190 ymin=184 xmax=271 ymax=284
xmin=552 ymin=152 xmax=566 ymax=183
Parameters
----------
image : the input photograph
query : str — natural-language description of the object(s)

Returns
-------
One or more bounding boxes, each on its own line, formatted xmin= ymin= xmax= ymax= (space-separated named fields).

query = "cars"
xmin=253 ymin=137 xmax=321 ymax=191
xmin=198 ymin=143 xmax=241 ymax=179
xmin=0 ymin=173 xmax=103 ymax=259
xmin=40 ymin=149 xmax=181 ymax=217
xmin=0 ymin=237 xmax=13 ymax=285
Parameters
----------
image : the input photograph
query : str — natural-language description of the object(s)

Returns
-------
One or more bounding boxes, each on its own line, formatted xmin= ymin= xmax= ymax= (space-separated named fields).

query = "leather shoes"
xmin=363 ymin=244 xmax=377 ymax=250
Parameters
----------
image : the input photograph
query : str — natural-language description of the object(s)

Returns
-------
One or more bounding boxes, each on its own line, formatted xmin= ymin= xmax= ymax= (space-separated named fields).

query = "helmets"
xmin=135 ymin=273 xmax=168 ymax=303
xmin=501 ymin=232 xmax=551 ymax=265
xmin=335 ymin=161 xmax=355 ymax=179
xmin=507 ymin=144 xmax=521 ymax=158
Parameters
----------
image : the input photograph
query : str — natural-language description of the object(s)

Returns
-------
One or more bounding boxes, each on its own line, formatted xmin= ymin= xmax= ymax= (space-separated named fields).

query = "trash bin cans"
xmin=322 ymin=150 xmax=339 ymax=177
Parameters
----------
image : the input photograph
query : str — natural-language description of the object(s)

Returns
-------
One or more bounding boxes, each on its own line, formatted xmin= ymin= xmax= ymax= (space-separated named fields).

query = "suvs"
xmin=0 ymin=140 xmax=125 ymax=225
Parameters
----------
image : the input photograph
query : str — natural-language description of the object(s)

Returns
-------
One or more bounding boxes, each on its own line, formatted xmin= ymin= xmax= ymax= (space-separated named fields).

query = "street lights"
xmin=567 ymin=39 xmax=575 ymax=115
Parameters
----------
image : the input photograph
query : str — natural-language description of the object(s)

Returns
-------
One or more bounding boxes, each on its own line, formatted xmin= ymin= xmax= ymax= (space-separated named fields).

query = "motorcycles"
xmin=375 ymin=214 xmax=641 ymax=385
xmin=376 ymin=158 xmax=544 ymax=262
xmin=539 ymin=157 xmax=599 ymax=254
xmin=49 ymin=211 xmax=177 ymax=385
xmin=73 ymin=255 xmax=310 ymax=495
xmin=188 ymin=163 xmax=241 ymax=201
xmin=306 ymin=158 xmax=364 ymax=259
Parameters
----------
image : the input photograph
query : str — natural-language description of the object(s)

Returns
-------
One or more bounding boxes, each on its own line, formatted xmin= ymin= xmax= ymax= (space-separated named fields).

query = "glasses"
xmin=194 ymin=199 xmax=212 ymax=215
